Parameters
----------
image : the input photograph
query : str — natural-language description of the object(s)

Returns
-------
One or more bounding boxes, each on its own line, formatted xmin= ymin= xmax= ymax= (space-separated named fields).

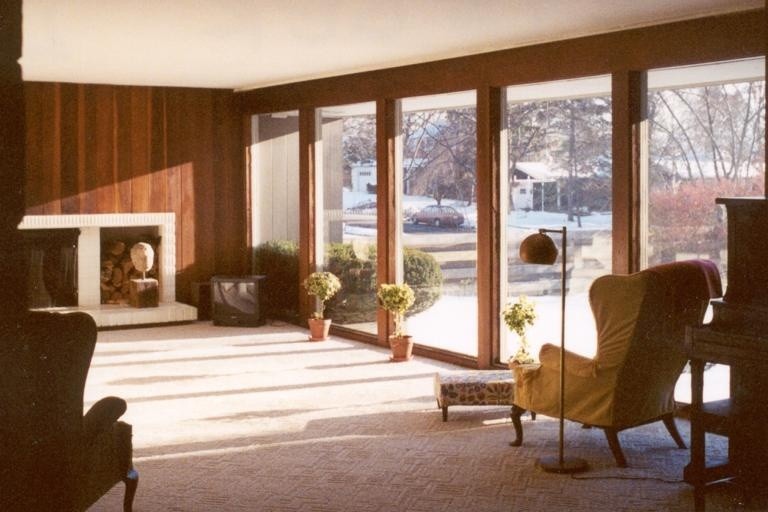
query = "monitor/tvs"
xmin=209 ymin=273 xmax=266 ymax=327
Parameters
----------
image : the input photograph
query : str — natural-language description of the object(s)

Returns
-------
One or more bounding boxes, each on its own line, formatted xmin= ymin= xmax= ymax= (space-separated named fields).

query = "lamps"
xmin=520 ymin=226 xmax=589 ymax=474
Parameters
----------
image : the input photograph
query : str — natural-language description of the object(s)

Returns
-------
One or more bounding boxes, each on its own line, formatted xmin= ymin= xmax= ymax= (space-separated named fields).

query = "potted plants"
xmin=304 ymin=271 xmax=342 ymax=340
xmin=502 ymin=295 xmax=536 ymax=369
xmin=377 ymin=279 xmax=416 ymax=360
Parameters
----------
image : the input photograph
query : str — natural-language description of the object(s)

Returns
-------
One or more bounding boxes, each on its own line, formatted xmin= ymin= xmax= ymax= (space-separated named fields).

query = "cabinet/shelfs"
xmin=675 ymin=197 xmax=768 ymax=512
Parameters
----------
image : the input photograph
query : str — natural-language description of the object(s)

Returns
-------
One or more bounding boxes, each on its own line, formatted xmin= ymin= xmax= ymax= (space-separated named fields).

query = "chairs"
xmin=0 ymin=312 xmax=138 ymax=512
xmin=508 ymin=259 xmax=722 ymax=469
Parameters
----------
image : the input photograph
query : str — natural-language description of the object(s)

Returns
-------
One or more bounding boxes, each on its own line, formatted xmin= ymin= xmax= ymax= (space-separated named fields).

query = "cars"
xmin=411 ymin=204 xmax=466 ymax=230
xmin=346 ymin=200 xmax=377 ymax=229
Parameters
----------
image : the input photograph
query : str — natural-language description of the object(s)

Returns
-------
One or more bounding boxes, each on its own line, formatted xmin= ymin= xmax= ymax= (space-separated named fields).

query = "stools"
xmin=434 ymin=370 xmax=536 ymax=420
